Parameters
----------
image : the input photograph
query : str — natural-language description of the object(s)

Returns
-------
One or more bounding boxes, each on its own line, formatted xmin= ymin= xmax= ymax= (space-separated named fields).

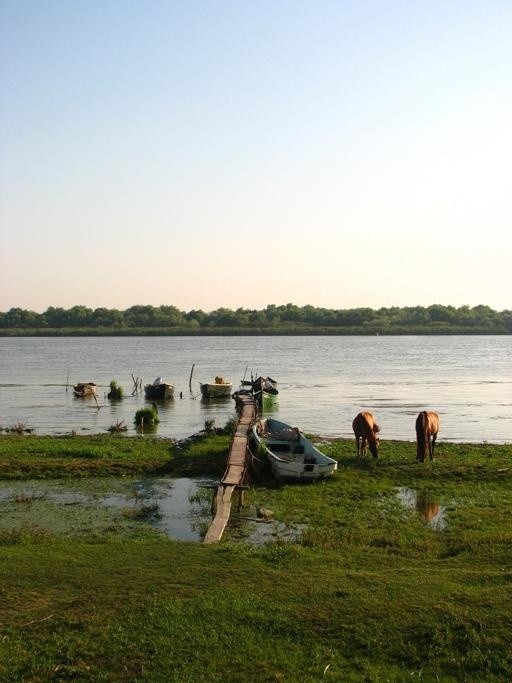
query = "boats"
xmin=144 ymin=376 xmax=174 ymax=401
xmin=249 ymin=416 xmax=339 ymax=490
xmin=201 ymin=370 xmax=279 ymax=414
xmin=74 ymin=382 xmax=97 ymax=398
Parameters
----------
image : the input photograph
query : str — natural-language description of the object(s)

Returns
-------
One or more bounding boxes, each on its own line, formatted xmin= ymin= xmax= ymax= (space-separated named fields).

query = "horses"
xmin=352 ymin=411 xmax=380 ymax=459
xmin=415 ymin=411 xmax=440 ymax=462
